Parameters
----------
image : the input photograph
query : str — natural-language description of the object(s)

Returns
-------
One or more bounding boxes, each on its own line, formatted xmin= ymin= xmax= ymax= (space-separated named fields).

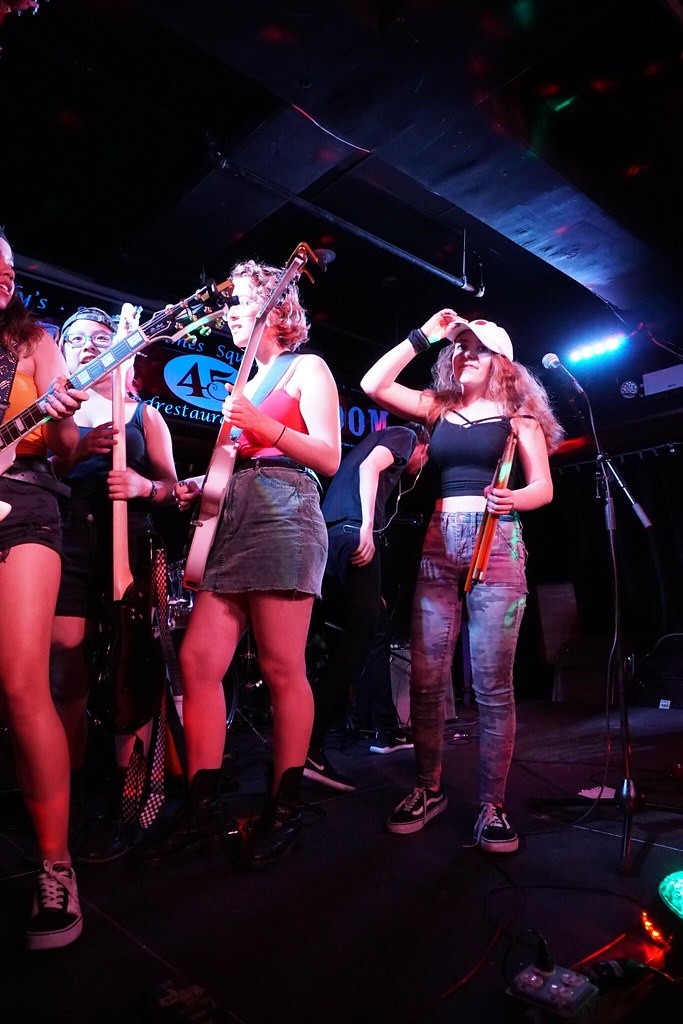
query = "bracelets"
xmin=407 ymin=327 xmax=430 ymax=354
xmin=273 ymin=425 xmax=286 ymax=446
xmin=146 ymin=481 xmax=158 ymax=498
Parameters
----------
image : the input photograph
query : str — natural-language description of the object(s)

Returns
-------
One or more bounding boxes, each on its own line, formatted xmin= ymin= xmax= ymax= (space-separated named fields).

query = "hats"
xmin=61 ymin=307 xmax=117 ymax=332
xmin=446 ymin=320 xmax=513 ymax=364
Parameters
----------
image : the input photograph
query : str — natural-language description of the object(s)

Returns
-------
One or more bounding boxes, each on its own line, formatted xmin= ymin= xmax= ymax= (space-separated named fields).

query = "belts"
xmin=1 ymin=456 xmax=73 ymax=498
xmin=231 ymin=457 xmax=324 ymax=495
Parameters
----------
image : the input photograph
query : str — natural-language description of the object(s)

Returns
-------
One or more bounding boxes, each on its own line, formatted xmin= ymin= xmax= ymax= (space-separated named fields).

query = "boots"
xmin=240 ymin=764 xmax=306 ymax=869
xmin=132 ymin=768 xmax=224 ymax=866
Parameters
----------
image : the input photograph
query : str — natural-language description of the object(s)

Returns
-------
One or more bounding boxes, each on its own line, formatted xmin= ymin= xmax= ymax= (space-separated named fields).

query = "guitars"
xmin=0 ymin=276 xmax=235 ymax=515
xmin=182 ymin=240 xmax=308 ymax=590
xmin=111 ymin=301 xmax=169 ymax=730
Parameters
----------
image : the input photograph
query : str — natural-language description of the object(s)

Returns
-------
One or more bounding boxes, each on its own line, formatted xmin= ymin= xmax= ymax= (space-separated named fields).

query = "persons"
xmin=135 ymin=241 xmax=340 ymax=872
xmin=0 ymin=225 xmax=178 ymax=951
xmin=322 ymin=308 xmax=554 ymax=853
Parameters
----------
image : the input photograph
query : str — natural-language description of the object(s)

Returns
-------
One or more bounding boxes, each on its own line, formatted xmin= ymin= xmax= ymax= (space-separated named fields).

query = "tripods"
xmin=527 ymin=400 xmax=683 ymax=876
xmin=326 ymin=681 xmax=390 ymax=755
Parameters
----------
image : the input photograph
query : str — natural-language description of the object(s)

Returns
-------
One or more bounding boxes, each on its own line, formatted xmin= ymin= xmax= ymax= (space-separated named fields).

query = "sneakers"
xmin=385 ymin=783 xmax=449 ymax=834
xmin=301 ymin=748 xmax=358 ymax=792
xmin=369 ymin=728 xmax=416 ymax=753
xmin=461 ymin=801 xmax=520 ymax=851
xmin=26 ymin=859 xmax=83 ymax=948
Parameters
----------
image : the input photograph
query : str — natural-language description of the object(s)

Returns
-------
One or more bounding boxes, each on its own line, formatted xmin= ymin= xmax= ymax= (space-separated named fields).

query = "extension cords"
xmin=505 ymin=964 xmax=601 ymax=1017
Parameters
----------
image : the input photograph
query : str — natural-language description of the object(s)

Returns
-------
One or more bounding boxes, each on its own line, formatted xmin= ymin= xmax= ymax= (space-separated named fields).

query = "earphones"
xmin=421 ymin=452 xmax=426 ymax=458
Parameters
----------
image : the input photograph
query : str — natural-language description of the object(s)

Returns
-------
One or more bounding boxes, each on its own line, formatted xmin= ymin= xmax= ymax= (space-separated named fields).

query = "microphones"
xmin=542 ymin=353 xmax=588 ymax=399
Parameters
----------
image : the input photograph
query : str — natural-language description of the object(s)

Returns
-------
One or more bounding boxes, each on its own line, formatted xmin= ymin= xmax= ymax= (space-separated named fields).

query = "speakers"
xmin=387 ymin=641 xmax=457 ymax=730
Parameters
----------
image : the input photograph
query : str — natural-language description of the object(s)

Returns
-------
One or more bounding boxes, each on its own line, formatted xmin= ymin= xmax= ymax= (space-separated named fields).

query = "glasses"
xmin=62 ymin=331 xmax=113 ymax=349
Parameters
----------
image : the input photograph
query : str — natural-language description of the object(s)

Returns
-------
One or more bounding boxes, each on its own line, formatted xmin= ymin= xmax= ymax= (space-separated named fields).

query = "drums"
xmin=137 ymin=617 xmax=239 ymax=739
xmin=168 ymin=555 xmax=205 ymax=615
